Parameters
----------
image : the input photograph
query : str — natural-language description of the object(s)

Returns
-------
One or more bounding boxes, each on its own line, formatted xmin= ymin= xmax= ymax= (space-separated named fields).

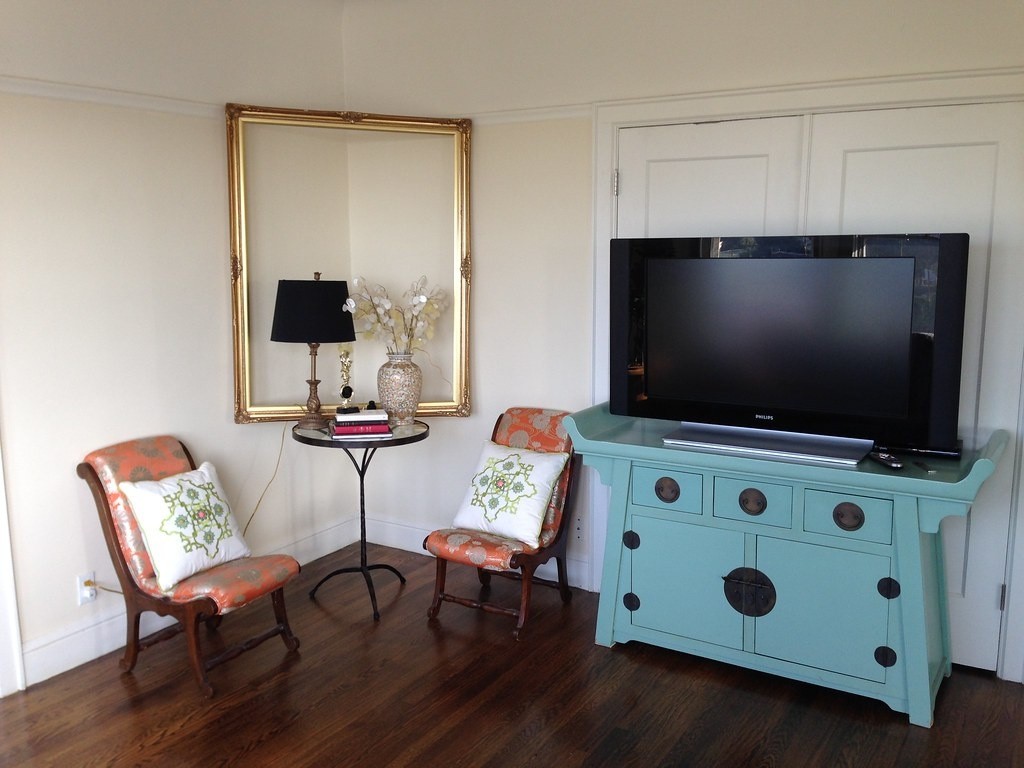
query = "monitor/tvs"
xmin=610 ymin=232 xmax=971 ymax=464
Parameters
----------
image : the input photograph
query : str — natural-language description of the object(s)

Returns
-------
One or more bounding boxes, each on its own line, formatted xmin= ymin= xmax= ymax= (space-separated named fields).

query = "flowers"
xmin=345 ymin=274 xmax=450 ymax=355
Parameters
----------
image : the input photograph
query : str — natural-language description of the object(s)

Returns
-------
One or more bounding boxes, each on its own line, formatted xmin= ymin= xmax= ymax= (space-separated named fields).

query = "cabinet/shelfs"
xmin=561 ymin=401 xmax=1010 ymax=729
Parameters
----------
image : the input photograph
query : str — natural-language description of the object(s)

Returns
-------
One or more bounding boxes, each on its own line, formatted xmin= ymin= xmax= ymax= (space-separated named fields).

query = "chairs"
xmin=423 ymin=406 xmax=584 ymax=643
xmin=75 ymin=433 xmax=302 ymax=698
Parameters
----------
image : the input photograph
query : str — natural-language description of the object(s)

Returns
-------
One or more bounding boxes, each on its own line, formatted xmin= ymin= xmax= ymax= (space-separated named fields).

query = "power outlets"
xmin=75 ymin=572 xmax=99 ymax=605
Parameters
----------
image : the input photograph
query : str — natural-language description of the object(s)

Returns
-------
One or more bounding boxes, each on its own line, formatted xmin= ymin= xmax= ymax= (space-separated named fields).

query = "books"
xmin=330 ymin=409 xmax=393 ymax=440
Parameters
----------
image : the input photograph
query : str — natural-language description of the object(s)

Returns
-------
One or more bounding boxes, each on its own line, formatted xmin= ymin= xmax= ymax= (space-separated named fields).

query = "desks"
xmin=291 ymin=421 xmax=430 ymax=626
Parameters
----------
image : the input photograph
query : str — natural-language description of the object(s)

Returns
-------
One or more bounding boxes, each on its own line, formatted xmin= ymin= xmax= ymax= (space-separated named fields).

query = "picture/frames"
xmin=224 ymin=100 xmax=472 ymax=418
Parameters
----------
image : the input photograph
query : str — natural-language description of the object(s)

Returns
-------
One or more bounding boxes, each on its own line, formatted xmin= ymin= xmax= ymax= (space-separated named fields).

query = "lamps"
xmin=269 ymin=271 xmax=358 ymax=429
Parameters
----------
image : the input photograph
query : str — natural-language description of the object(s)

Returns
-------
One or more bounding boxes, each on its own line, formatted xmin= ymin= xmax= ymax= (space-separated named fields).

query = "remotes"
xmin=870 ymin=451 xmax=903 ymax=468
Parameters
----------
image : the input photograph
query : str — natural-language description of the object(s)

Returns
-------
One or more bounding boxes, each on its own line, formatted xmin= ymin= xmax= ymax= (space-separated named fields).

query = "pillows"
xmin=452 ymin=439 xmax=570 ymax=552
xmin=119 ymin=461 xmax=252 ymax=593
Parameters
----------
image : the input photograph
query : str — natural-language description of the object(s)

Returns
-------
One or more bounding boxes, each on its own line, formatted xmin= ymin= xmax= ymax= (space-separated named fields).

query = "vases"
xmin=376 ymin=352 xmax=424 ymax=425
